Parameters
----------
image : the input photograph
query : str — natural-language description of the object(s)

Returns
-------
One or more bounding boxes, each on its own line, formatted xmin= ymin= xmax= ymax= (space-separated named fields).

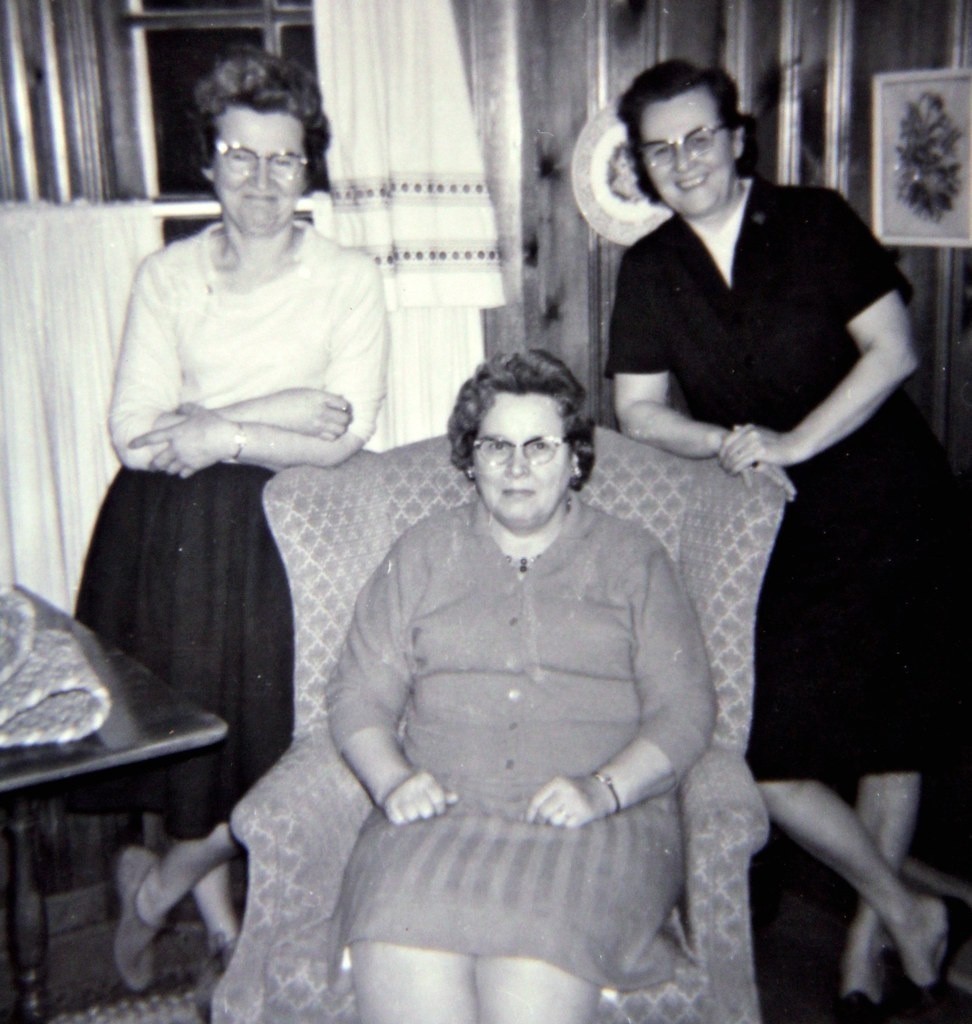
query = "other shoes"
xmin=832 ymin=940 xmax=887 ymax=1024
xmin=116 ymin=846 xmax=167 ymax=992
xmin=883 ymin=893 xmax=972 ymax=1016
xmin=194 ymin=941 xmax=234 ymax=1024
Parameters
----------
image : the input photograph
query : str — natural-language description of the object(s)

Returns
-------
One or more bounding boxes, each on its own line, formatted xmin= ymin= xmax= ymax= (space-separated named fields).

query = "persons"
xmin=603 ymin=59 xmax=953 ymax=1024
xmin=326 ymin=351 xmax=719 ymax=1024
xmin=73 ymin=53 xmax=387 ymax=989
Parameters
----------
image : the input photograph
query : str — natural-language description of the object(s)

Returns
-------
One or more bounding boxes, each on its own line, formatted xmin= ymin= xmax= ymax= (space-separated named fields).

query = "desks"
xmin=0 ymin=583 xmax=230 ymax=1022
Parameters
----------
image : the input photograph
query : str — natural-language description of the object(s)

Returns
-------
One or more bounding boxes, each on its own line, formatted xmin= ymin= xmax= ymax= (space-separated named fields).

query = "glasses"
xmin=210 ymin=139 xmax=310 ymax=185
xmin=472 ymin=434 xmax=566 ymax=470
xmin=639 ymin=123 xmax=727 ymax=168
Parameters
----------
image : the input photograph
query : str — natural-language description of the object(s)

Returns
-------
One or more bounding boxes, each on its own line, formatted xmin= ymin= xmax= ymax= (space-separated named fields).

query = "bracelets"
xmin=233 ymin=423 xmax=243 ymax=461
xmin=592 ymin=770 xmax=621 ymax=813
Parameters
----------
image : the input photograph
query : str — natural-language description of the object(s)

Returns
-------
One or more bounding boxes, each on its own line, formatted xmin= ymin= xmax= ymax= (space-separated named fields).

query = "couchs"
xmin=203 ymin=428 xmax=795 ymax=1022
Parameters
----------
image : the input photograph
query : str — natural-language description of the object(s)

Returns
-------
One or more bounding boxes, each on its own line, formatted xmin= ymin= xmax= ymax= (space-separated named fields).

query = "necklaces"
xmin=505 ymin=498 xmax=571 ymax=573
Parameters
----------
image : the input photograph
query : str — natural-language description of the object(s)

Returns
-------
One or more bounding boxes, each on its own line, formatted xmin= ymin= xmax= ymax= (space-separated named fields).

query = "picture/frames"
xmin=571 ymin=103 xmax=672 ymax=247
xmin=869 ymin=70 xmax=972 ymax=247
